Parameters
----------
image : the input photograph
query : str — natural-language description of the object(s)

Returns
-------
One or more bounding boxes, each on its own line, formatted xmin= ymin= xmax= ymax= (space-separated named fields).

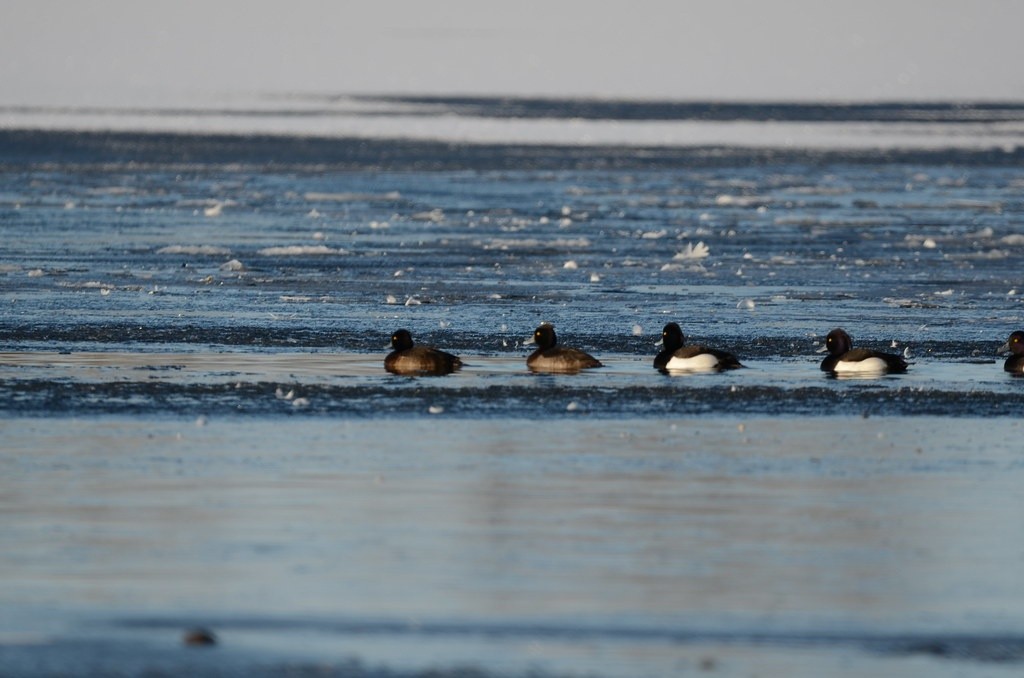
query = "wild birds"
xmin=997 ymin=331 xmax=1023 ymax=375
xmin=815 ymin=327 xmax=910 ymax=378
xmin=654 ymin=321 xmax=740 ymax=376
xmin=521 ymin=325 xmax=604 ymax=372
xmin=382 ymin=328 xmax=463 ymax=373
